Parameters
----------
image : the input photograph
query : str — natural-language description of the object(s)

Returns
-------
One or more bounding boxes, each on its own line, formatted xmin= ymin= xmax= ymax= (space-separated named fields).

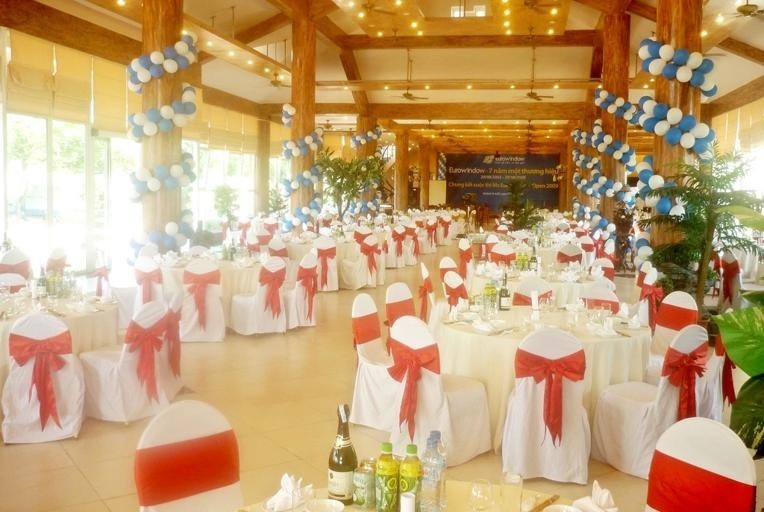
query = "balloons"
xmin=282 ymin=163 xmax=323 ymax=232
xmin=572 ymin=37 xmax=717 ymax=287
xmin=125 ymin=30 xmax=200 ymax=142
xmin=282 ymin=127 xmax=323 ymax=159
xmin=349 ymin=125 xmax=382 ymax=213
xmin=280 ymin=102 xmax=296 ymax=122
xmin=131 ymin=208 xmax=196 ymax=254
xmin=436 ymin=150 xmax=447 ymax=181
xmin=126 ymin=152 xmax=197 ymax=201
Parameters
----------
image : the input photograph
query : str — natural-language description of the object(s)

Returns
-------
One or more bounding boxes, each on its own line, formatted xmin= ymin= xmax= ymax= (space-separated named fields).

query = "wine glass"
xmin=538 ymin=296 xmax=612 ymax=331
xmin=479 ymin=259 xmax=487 ymax=279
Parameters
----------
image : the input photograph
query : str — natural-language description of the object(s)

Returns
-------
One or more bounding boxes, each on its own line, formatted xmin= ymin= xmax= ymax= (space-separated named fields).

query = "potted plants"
xmin=638 ymin=135 xmax=764 ymax=331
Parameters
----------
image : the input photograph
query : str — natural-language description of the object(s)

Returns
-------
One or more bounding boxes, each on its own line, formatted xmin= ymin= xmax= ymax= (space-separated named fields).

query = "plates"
xmin=543 ymin=503 xmax=583 ymax=512
xmin=307 ymin=498 xmax=344 ymax=511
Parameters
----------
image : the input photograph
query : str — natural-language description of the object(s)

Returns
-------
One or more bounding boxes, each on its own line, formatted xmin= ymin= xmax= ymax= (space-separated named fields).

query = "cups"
xmin=467 ymin=470 xmax=523 ymax=511
xmin=475 ymin=295 xmax=498 ymax=322
xmin=601 ymin=317 xmax=622 ymax=332
xmin=0 ymin=288 xmax=33 ymax=319
xmin=38 ymin=293 xmax=58 ymax=313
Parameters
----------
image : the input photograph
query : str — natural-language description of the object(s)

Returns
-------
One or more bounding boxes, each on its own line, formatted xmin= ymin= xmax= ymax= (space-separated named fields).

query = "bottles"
xmin=390 ymin=213 xmax=395 ymax=224
xmin=374 ymin=429 xmax=447 ymax=512
xmin=37 ymin=263 xmax=74 ymax=300
xmin=483 ymin=273 xmax=497 ymax=310
xmin=499 ymin=273 xmax=512 ymax=310
xmin=328 ymin=404 xmax=357 ymax=506
xmin=221 ymin=237 xmax=237 ymax=261
xmin=334 ymin=224 xmax=346 ymax=241
xmin=515 ymin=246 xmax=538 ymax=273
xmin=370 ymin=217 xmax=375 ymax=229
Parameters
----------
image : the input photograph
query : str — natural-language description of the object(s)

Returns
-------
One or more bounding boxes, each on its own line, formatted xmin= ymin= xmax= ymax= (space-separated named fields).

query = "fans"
xmin=520 ymin=120 xmax=541 ymax=156
xmin=717 ymin=0 xmax=764 ymax=21
xmin=259 ymin=42 xmax=291 ymax=87
xmin=702 ymin=51 xmax=725 ymax=57
xmin=388 ymin=45 xmax=429 ymax=100
xmin=417 ymin=119 xmax=476 ymax=155
xmin=628 ymin=54 xmax=655 ymax=83
xmin=510 ymin=45 xmax=554 ymax=101
xmin=320 ymin=120 xmax=342 ymax=131
